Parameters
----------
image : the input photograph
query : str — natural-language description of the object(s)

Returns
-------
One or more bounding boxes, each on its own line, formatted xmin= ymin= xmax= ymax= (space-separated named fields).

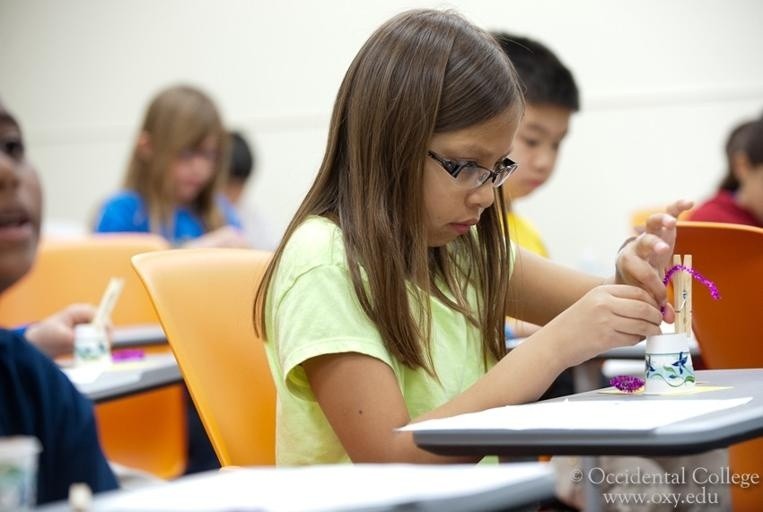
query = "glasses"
xmin=427 ymin=149 xmax=518 ymax=190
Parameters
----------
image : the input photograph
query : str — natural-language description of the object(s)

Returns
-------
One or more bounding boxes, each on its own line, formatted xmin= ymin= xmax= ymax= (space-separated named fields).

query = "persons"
xmin=487 ymin=30 xmax=731 ymax=511
xmin=683 ymin=111 xmax=763 ymax=228
xmin=221 ymin=132 xmax=251 ymax=206
xmin=4 ymin=302 xmax=110 ymax=360
xmin=89 ymin=84 xmax=255 ymax=252
xmin=252 ymin=9 xmax=695 ymax=510
xmin=0 ymin=101 xmax=121 ymax=506
